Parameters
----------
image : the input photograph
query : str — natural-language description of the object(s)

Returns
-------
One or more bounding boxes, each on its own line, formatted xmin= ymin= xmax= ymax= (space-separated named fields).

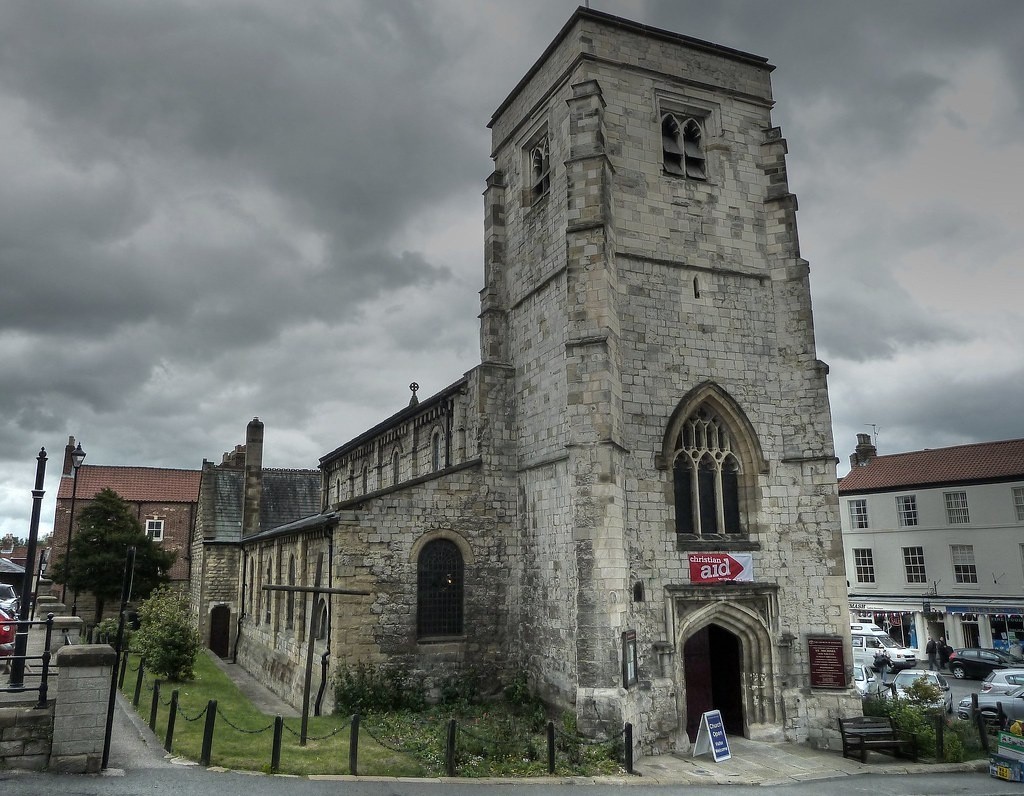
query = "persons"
xmin=873 ymin=644 xmax=891 ymax=685
xmin=1008 ymin=638 xmax=1023 ymax=660
xmin=937 ymin=636 xmax=950 ymax=670
xmin=926 ymin=636 xmax=940 ymax=672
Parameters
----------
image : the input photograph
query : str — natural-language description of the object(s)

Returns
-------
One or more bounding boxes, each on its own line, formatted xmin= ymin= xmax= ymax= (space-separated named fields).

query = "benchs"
xmin=839 ymin=716 xmax=919 ymax=764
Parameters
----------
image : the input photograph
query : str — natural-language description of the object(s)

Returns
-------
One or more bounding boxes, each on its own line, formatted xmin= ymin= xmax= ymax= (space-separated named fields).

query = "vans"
xmin=851 ymin=630 xmax=917 ymax=674
xmin=850 ymin=623 xmax=900 ymax=647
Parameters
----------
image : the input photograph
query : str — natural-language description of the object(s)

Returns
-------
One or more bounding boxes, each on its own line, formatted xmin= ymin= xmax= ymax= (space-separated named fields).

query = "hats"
xmin=879 ymin=644 xmax=884 ymax=648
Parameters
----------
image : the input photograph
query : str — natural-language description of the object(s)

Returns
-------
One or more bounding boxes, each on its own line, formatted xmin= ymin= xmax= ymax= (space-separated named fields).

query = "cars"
xmin=979 ymin=669 xmax=1024 ymax=695
xmin=854 ymin=663 xmax=880 ymax=698
xmin=0 ymin=584 xmax=21 ymax=618
xmin=0 ymin=608 xmax=16 ymax=656
xmin=957 ymin=685 xmax=1024 ymax=725
xmin=882 ymin=669 xmax=953 ymax=716
xmin=948 ymin=648 xmax=1024 ymax=679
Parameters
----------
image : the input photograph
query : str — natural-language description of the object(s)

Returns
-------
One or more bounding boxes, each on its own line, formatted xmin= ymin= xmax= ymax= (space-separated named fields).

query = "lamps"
xmin=438 ymin=573 xmax=454 ymax=593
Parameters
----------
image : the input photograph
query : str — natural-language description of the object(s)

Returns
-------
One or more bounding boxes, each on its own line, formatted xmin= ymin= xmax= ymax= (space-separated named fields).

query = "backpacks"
xmin=873 ymin=656 xmax=889 ymax=667
xmin=936 ymin=641 xmax=941 ymax=649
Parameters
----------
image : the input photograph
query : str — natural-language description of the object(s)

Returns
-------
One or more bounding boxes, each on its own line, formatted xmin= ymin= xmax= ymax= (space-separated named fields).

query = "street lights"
xmin=30 ymin=549 xmax=48 ymax=628
xmin=62 ymin=441 xmax=86 ymax=603
